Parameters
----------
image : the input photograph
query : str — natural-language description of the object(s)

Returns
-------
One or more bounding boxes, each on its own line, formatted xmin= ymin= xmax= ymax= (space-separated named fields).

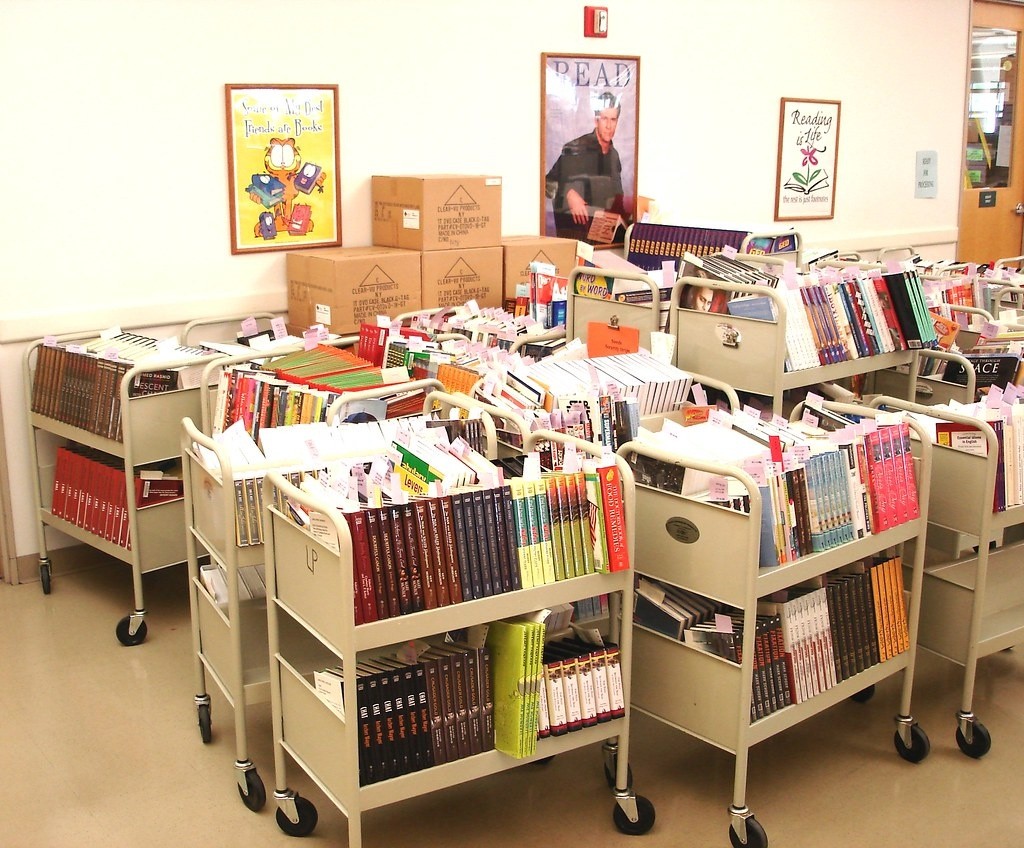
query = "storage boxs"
xmin=286 ymin=247 xmax=422 ymax=336
xmin=422 ymin=247 xmax=504 ymax=309
xmin=501 ymin=235 xmax=578 ymax=301
xmin=371 ymin=176 xmax=503 ymax=251
xmin=966 ymin=119 xmax=992 ymax=187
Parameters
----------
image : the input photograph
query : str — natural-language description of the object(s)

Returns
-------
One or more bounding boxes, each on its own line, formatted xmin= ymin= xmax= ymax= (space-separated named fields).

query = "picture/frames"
xmin=224 ymin=84 xmax=342 ymax=254
xmin=774 ymin=97 xmax=841 ymax=223
xmin=540 ymin=52 xmax=640 ymax=250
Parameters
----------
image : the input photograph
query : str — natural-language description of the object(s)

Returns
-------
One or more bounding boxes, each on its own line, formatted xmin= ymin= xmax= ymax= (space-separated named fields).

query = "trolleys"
xmin=21 ymin=222 xmax=1024 ymax=846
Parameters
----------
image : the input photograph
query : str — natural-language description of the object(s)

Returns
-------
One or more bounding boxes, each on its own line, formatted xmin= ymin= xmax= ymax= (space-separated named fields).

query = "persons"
xmin=680 ymin=284 xmax=714 ymax=312
xmin=545 ymin=92 xmax=625 ymax=244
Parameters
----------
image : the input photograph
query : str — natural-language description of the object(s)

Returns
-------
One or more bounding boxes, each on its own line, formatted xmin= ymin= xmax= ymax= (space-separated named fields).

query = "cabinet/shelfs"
xmin=24 ymin=231 xmax=1024 ymax=848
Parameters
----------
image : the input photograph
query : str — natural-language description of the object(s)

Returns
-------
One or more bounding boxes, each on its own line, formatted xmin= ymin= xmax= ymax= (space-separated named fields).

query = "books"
xmin=586 ymin=210 xmax=626 ymax=244
xmin=31 ymin=222 xmax=1024 ymax=787
